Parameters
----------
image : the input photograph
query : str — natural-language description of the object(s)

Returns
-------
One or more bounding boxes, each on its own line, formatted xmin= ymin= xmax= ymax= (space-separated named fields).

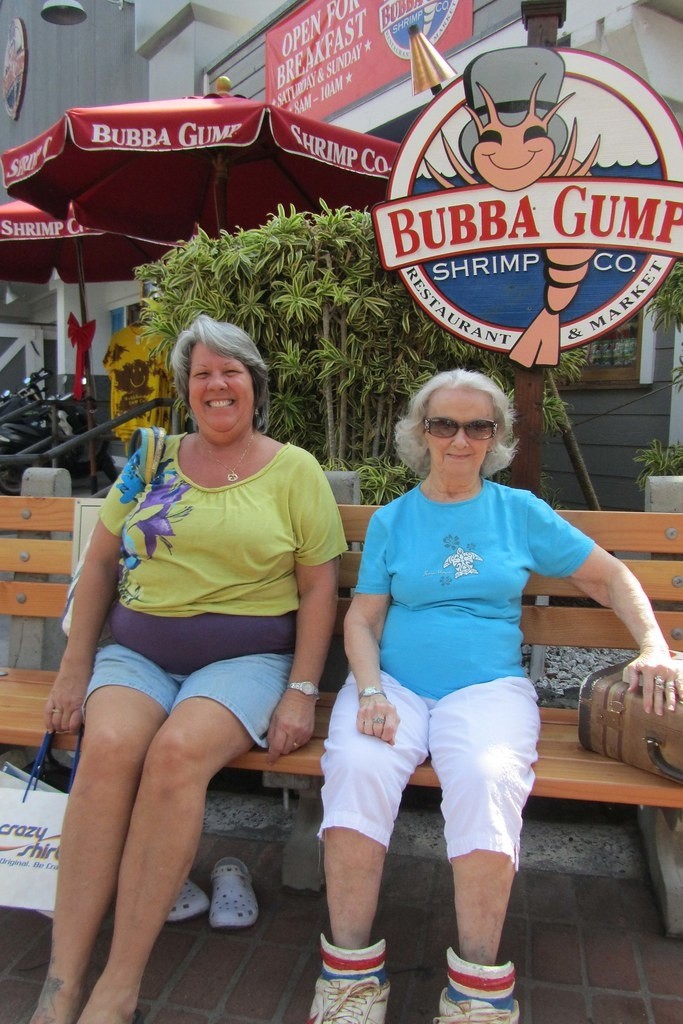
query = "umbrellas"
xmin=0 ymin=76 xmax=401 ymax=245
xmin=0 ymin=199 xmax=175 ymax=494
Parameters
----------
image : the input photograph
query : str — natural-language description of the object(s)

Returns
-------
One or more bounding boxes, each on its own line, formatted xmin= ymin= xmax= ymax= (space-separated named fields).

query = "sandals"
xmin=209 ymin=857 xmax=259 ymax=929
xmin=165 ymin=878 xmax=208 ymax=922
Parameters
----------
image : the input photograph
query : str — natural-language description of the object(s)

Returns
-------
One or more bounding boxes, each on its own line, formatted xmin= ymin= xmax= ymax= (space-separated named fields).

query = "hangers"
xmin=112 ymin=306 xmax=162 ymax=339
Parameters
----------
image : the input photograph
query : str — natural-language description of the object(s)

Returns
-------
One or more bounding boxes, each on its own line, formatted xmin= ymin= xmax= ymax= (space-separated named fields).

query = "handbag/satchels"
xmin=1 ymin=723 xmax=86 ymax=910
xmin=62 ymin=426 xmax=165 ymax=639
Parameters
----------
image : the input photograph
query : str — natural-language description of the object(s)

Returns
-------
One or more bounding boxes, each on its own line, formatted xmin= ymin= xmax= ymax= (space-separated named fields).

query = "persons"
xmin=308 ymin=368 xmax=683 ymax=1024
xmin=29 ymin=314 xmax=348 ymax=1024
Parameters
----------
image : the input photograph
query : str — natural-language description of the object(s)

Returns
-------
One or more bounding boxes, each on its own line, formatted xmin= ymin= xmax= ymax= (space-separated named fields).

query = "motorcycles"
xmin=0 ymin=369 xmax=119 ymax=496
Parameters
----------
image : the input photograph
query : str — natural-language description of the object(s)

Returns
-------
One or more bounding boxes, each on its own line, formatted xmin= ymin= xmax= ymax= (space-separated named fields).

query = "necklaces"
xmin=199 ymin=434 xmax=253 ymax=481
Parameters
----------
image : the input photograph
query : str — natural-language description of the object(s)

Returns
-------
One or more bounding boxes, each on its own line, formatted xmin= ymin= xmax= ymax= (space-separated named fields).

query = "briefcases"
xmin=579 ymin=654 xmax=683 ymax=781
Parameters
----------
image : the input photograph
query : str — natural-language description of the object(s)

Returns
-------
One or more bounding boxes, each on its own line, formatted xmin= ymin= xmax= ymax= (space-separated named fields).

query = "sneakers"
xmin=308 ymin=973 xmax=390 ymax=1024
xmin=434 ymin=988 xmax=519 ymax=1024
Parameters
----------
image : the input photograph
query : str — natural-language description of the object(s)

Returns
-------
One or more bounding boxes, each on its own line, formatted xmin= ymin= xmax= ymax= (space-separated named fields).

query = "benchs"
xmin=0 ymin=496 xmax=683 ymax=939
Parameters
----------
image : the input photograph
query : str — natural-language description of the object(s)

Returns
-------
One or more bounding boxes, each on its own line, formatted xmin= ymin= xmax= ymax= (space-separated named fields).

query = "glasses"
xmin=424 ymin=417 xmax=498 ymax=440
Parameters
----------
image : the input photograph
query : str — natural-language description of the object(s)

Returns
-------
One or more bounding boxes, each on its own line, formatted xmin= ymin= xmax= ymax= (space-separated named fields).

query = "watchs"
xmin=359 ymin=687 xmax=387 ymax=700
xmin=287 ymin=681 xmax=319 ymax=696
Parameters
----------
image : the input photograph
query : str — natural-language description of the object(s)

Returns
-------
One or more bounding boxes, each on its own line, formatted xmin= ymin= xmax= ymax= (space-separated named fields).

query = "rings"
xmin=373 ymin=717 xmax=385 ymax=723
xmin=655 ymin=676 xmax=663 ymax=685
xmin=294 ymin=742 xmax=299 ymax=748
xmin=52 ymin=708 xmax=60 ymax=712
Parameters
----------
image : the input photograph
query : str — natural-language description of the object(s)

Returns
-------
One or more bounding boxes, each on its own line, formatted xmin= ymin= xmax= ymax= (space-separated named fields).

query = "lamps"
xmin=40 ymin=0 xmax=135 ymax=25
